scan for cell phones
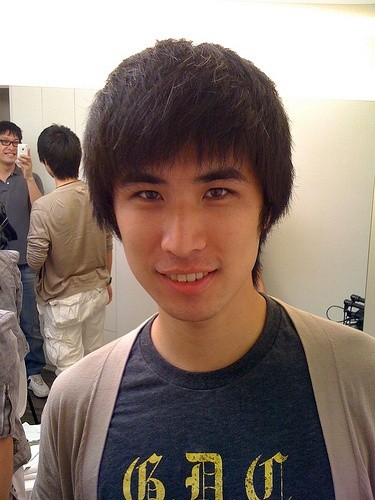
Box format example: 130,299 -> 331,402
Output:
18,144 -> 28,158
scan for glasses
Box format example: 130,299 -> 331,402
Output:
0,139 -> 21,147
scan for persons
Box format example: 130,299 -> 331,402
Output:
26,125 -> 113,376
30,40 -> 375,500
0,228 -> 36,324
0,121 -> 53,399
0,310 -> 31,498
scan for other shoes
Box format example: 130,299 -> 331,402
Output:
27,374 -> 49,397
